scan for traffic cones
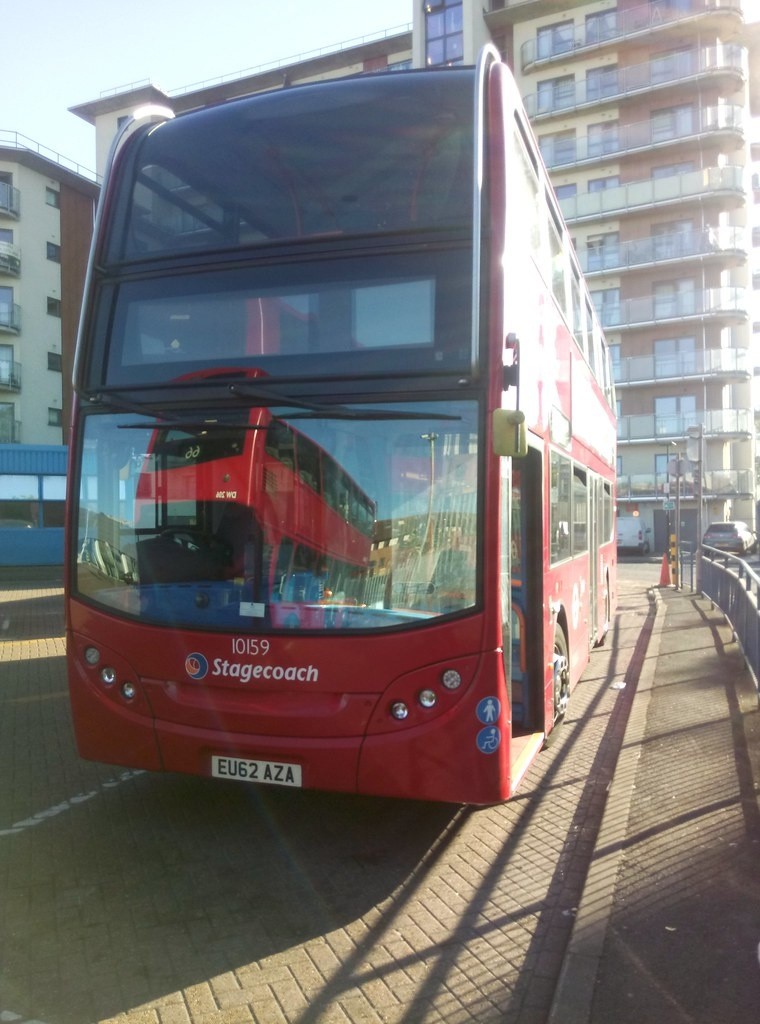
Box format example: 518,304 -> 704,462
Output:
658,549 -> 673,588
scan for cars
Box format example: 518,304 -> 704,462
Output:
702,521 -> 758,557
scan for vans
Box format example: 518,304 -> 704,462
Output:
613,517 -> 652,557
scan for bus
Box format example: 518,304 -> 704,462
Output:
133,365 -> 378,615
216,295 -> 369,355
61,43 -> 620,805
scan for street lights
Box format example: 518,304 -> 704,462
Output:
406,430 -> 443,598
